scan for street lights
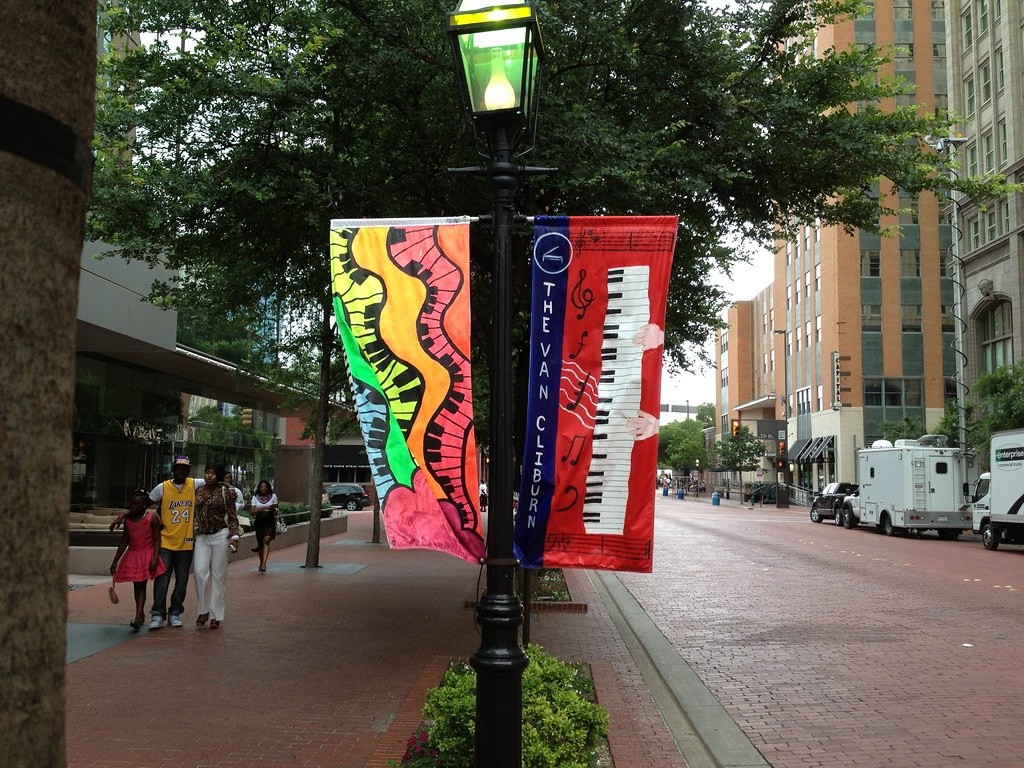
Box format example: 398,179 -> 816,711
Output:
447,0 -> 561,768
774,330 -> 789,509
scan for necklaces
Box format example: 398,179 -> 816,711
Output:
142,513 -> 146,519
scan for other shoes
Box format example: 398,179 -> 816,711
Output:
258,562 -> 267,573
130,616 -> 145,629
196,614 -> 209,626
211,619 -> 220,627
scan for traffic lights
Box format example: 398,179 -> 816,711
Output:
778,461 -> 786,468
779,442 -> 785,456
732,421 -> 740,437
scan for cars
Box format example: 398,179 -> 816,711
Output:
324,483 -> 370,511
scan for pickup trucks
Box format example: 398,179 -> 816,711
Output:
809,483 -> 859,525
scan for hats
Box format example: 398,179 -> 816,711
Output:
173,455 -> 192,466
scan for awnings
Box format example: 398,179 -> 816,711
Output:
788,435 -> 834,464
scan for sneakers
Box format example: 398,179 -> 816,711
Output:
149,615 -> 164,629
168,616 -> 184,627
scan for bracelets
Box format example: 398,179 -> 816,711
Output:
228,487 -> 235,490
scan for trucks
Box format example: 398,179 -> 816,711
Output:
962,426 -> 1024,550
842,434 -> 973,540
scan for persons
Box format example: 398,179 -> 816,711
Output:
479,479 -> 489,512
109,455 -> 244,630
250,480 -> 278,572
656,473 -> 763,511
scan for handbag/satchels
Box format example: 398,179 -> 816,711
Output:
224,513 -> 244,536
275,507 -> 288,536
108,587 -> 119,604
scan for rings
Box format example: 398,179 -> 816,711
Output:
233,497 -> 234,498
